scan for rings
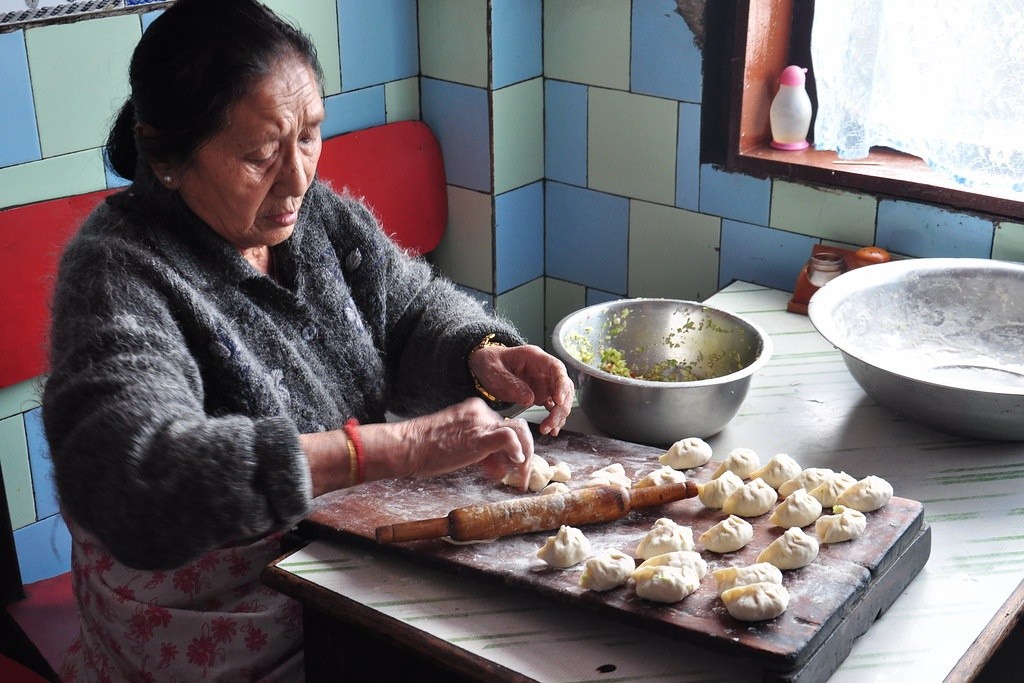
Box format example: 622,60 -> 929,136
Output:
504,416 -> 510,420
545,396 -> 555,409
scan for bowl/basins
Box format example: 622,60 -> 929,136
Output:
808,256 -> 1024,443
551,296 -> 775,447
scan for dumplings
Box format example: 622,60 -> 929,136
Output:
499,437 -> 894,621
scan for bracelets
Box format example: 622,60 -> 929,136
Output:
342,419 -> 364,487
468,333 -> 507,403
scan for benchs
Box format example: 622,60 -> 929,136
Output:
0,120 -> 449,683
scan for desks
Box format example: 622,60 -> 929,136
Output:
265,276 -> 1024,683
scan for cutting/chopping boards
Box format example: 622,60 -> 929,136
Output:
305,418 -> 931,683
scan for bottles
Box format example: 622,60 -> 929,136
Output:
770,61 -> 813,152
808,251 -> 847,288
854,246 -> 890,270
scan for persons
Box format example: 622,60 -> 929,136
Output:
41,0 -> 575,683
